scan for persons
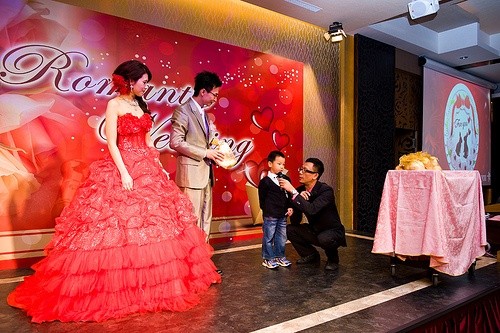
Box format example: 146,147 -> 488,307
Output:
7,60 -> 223,324
258,151 -> 348,270
169,71 -> 225,283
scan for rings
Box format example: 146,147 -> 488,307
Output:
126,185 -> 130,188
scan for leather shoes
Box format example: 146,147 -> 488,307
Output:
297,252 -> 320,264
324,259 -> 339,272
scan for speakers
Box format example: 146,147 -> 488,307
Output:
408,0 -> 440,20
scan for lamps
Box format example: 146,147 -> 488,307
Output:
324,22 -> 347,42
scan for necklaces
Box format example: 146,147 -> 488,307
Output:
117,93 -> 139,111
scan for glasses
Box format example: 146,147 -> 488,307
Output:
208,90 -> 218,98
298,167 -> 317,174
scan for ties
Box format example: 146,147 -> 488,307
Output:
201,109 -> 208,137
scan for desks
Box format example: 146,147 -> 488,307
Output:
371,170 -> 488,285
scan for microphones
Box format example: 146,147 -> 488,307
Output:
278,172 -> 284,191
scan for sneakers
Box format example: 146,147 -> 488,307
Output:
262,258 -> 280,269
274,256 -> 292,267
